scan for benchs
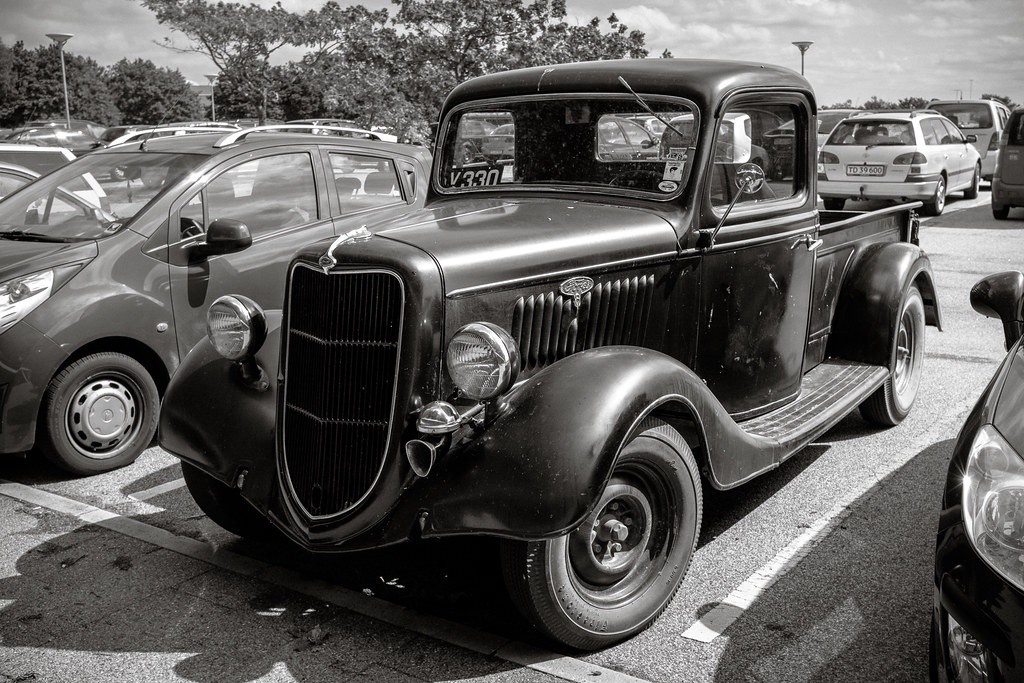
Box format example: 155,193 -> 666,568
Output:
335,173 -> 395,211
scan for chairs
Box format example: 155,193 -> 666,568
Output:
198,172 -> 235,202
854,127 -> 888,140
947,116 -> 958,126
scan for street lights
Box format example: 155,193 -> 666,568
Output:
203,74 -> 220,122
792,41 -> 814,76
45,33 -> 74,129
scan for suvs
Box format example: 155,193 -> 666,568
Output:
816,109 -> 982,217
0,126 -> 431,474
925,97 -> 1011,187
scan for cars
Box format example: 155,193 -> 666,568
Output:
597,116 -> 661,161
761,109 -> 879,182
598,114 -> 622,139
278,119 -> 389,173
620,117 -> 667,139
158,59 -> 943,652
928,269 -> 1024,683
991,105 -> 1024,220
658,108 -> 787,188
0,119 -> 121,225
481,124 -> 515,165
410,119 -> 498,164
92,119 -> 259,190
